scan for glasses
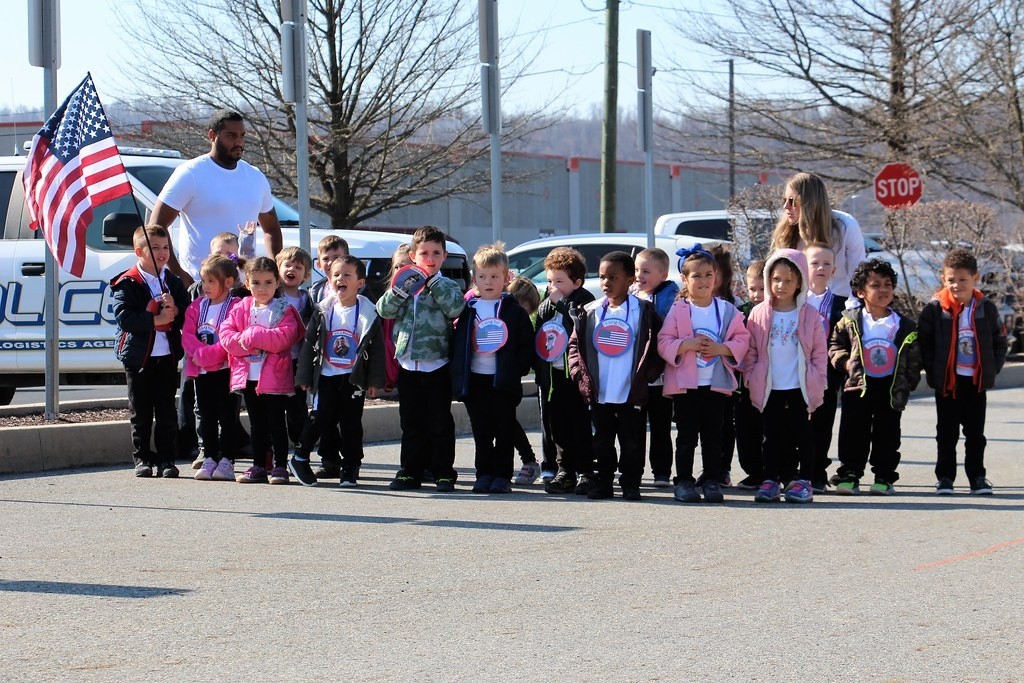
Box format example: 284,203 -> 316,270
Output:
783,197 -> 800,207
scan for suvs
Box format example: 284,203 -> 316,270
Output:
1,141 -> 469,406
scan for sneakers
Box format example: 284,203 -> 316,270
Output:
156,462 -> 178,478
837,479 -> 860,494
870,479 -> 895,494
936,477 -> 954,494
783,480 -> 813,503
190,448 -> 361,486
970,476 -> 993,494
472,469 -> 735,504
134,461 -> 152,476
754,480 -> 781,503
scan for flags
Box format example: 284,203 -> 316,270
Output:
21,74 -> 131,277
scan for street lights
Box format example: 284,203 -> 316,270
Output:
715,59 -> 735,208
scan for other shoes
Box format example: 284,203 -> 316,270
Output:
390,470 -> 422,490
810,479 -> 827,493
437,472 -> 454,491
738,471 -> 758,490
828,472 -> 838,484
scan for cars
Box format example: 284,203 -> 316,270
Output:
508,233 -> 731,300
865,233 -> 1024,351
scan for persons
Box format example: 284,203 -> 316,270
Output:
917,247 -> 1008,494
375,226 -> 466,491
110,225 -> 193,477
454,241 -> 848,503
148,109 -> 284,463
829,259 -> 923,496
235,221 -> 386,486
770,173 -> 867,308
181,232 -> 243,467
220,257 -> 307,485
181,253 -> 244,481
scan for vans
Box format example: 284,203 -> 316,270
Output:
657,209 -> 783,257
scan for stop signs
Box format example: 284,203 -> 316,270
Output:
872,162 -> 922,209
